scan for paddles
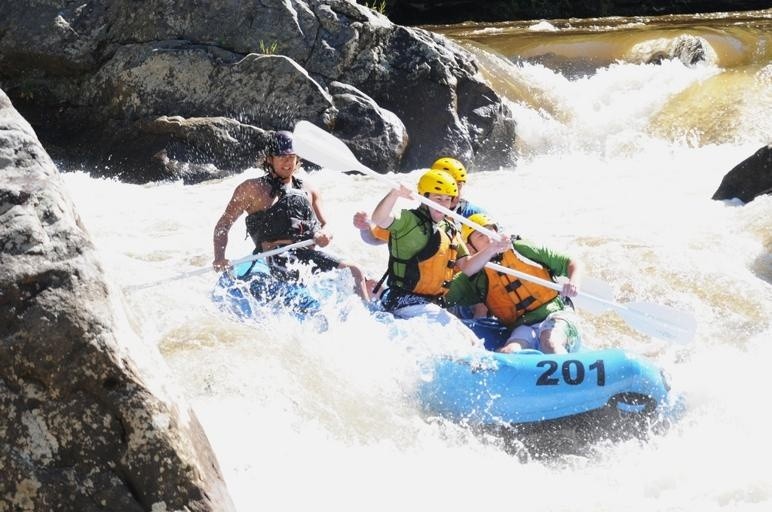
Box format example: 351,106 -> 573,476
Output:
291,120 -> 502,240
121,233 -> 334,294
485,261 -> 706,348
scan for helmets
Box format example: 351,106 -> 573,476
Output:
266,131 -> 295,157
463,212 -> 498,243
418,156 -> 467,198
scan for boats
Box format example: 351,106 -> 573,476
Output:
212,250 -> 664,427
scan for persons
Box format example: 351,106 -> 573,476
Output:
214,129 -> 386,308
371,167 -> 510,319
352,155 -> 486,274
443,213 -> 578,355
